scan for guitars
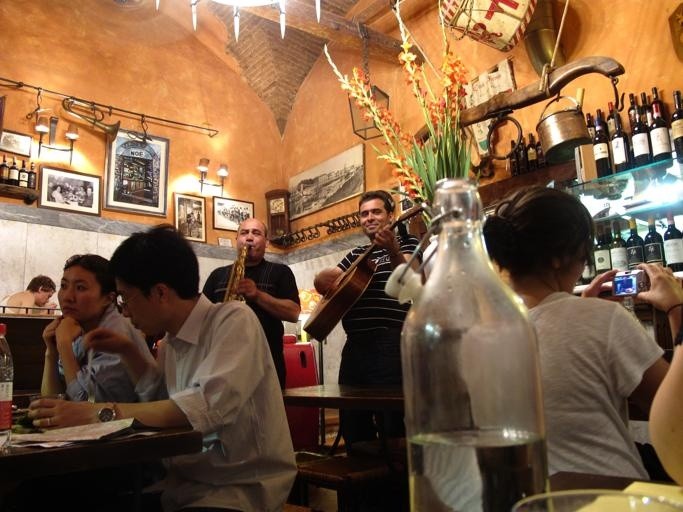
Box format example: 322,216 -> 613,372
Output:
303,200 -> 431,342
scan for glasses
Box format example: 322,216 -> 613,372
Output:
115,292 -> 140,308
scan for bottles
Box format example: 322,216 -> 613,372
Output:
507,138 -> 544,173
584,213 -> 681,273
0,153 -> 37,192
0,323 -> 14,449
584,87 -> 682,176
401,180 -> 552,511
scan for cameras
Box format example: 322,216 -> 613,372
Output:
613,269 -> 646,297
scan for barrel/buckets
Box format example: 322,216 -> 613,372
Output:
536,95 -> 592,166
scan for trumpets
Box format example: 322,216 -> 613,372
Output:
61,97 -> 122,144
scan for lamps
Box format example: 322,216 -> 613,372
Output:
33,115 -> 79,167
341,27 -> 391,139
196,157 -> 229,197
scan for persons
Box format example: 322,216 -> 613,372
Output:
1,275 -> 57,315
485,186 -> 683,511
619,277 -> 632,292
179,199 -> 202,237
47,175 -> 94,208
28,223 -> 297,511
203,218 -> 301,391
649,328 -> 683,488
314,190 -> 423,512
217,205 -> 250,225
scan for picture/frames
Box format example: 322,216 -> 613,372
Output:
102,125 -> 170,217
173,193 -> 206,243
287,141 -> 366,222
0,128 -> 33,157
211,195 -> 253,232
37,164 -> 101,217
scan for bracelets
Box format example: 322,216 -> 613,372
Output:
665,303 -> 682,312
673,327 -> 683,343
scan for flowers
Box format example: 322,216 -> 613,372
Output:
319,5 -> 479,229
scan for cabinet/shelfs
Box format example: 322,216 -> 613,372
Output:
565,154 -> 683,297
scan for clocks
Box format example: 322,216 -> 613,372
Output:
264,188 -> 290,241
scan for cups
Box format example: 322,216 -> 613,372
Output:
29,391 -> 67,431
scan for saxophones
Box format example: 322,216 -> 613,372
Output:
223,243 -> 251,304
